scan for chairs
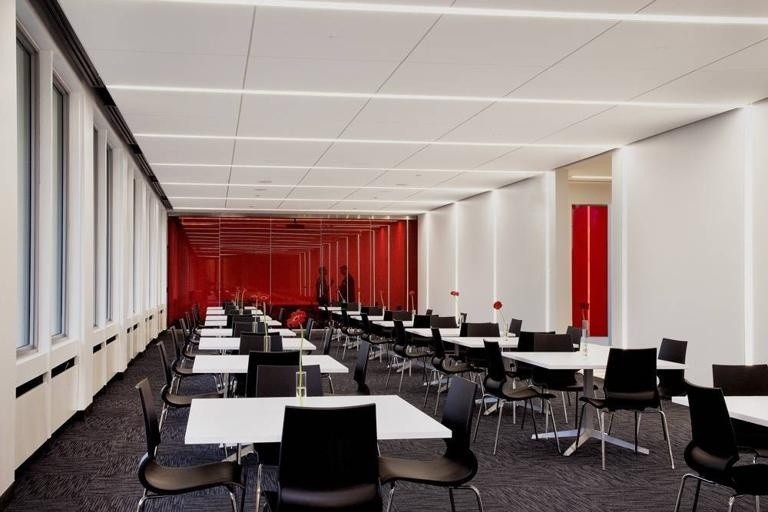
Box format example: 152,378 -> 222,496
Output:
244,363 -> 324,511
135,376 -> 251,511
153,302 -> 688,456
674,383 -> 767,512
575,347 -> 676,471
711,362 -> 767,464
378,374 -> 485,512
255,403 -> 383,511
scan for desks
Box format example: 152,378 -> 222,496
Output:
184,394 -> 454,467
722,395 -> 768,428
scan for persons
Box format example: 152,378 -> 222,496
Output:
315,266 -> 333,306
336,265 -> 356,303
